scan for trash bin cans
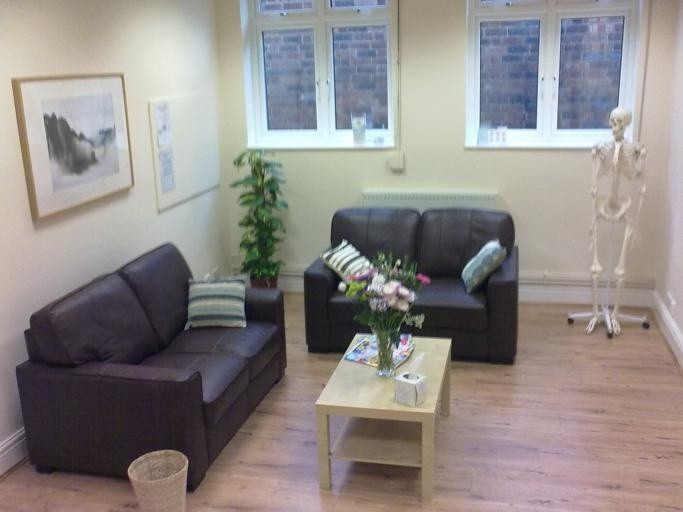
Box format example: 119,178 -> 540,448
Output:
127,450 -> 189,512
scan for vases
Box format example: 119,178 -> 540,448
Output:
372,329 -> 400,377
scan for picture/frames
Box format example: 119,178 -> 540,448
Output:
11,70 -> 136,221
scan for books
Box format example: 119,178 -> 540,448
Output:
344,333 -> 415,370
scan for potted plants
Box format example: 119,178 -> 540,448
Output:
228,147 -> 285,290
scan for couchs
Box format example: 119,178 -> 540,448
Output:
304,206 -> 518,364
15,287 -> 287,494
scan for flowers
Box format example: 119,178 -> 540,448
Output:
337,251 -> 432,348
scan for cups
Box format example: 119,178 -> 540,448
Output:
349,111 -> 367,145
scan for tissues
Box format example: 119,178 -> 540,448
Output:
393,353 -> 428,407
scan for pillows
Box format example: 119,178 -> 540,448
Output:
461,238 -> 508,294
184,273 -> 248,331
119,242 -> 194,349
320,237 -> 372,285
29,272 -> 162,367
418,208 -> 516,278
330,208 -> 422,273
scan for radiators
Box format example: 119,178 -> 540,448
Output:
362,188 -> 499,212
